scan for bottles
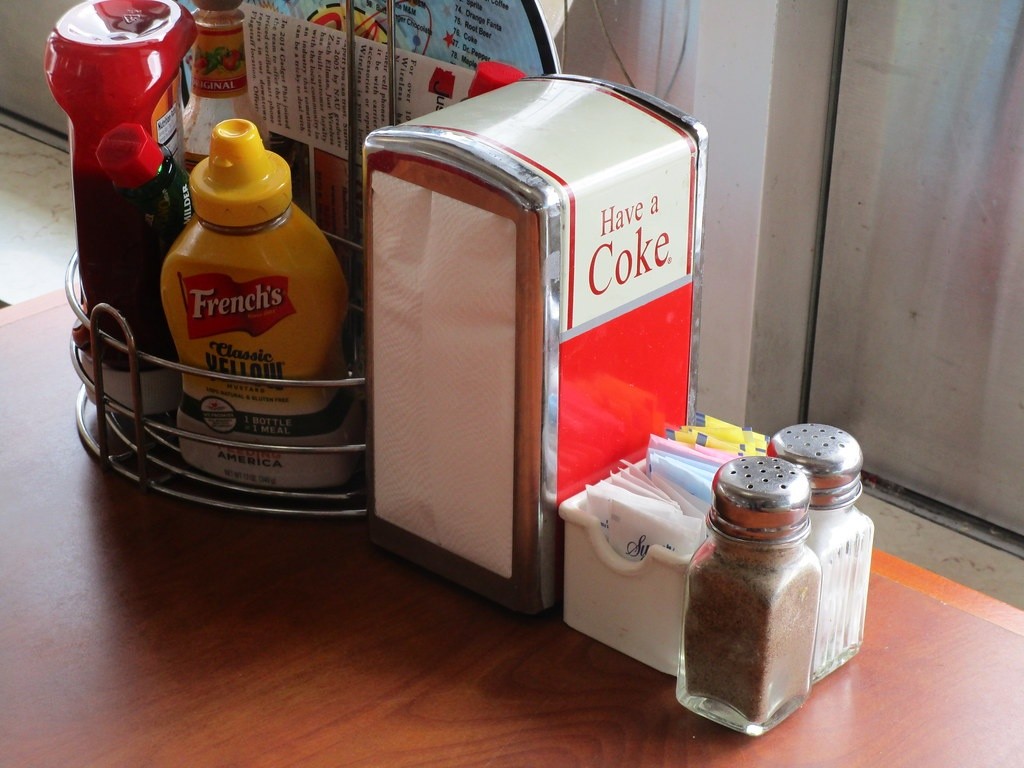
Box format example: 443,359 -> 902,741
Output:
182,0 -> 269,173
677,454 -> 823,734
44,0 -> 196,417
771,424 -> 875,680
160,118 -> 360,493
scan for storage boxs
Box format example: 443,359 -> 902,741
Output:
560,457 -> 688,676
363,72 -> 710,623
